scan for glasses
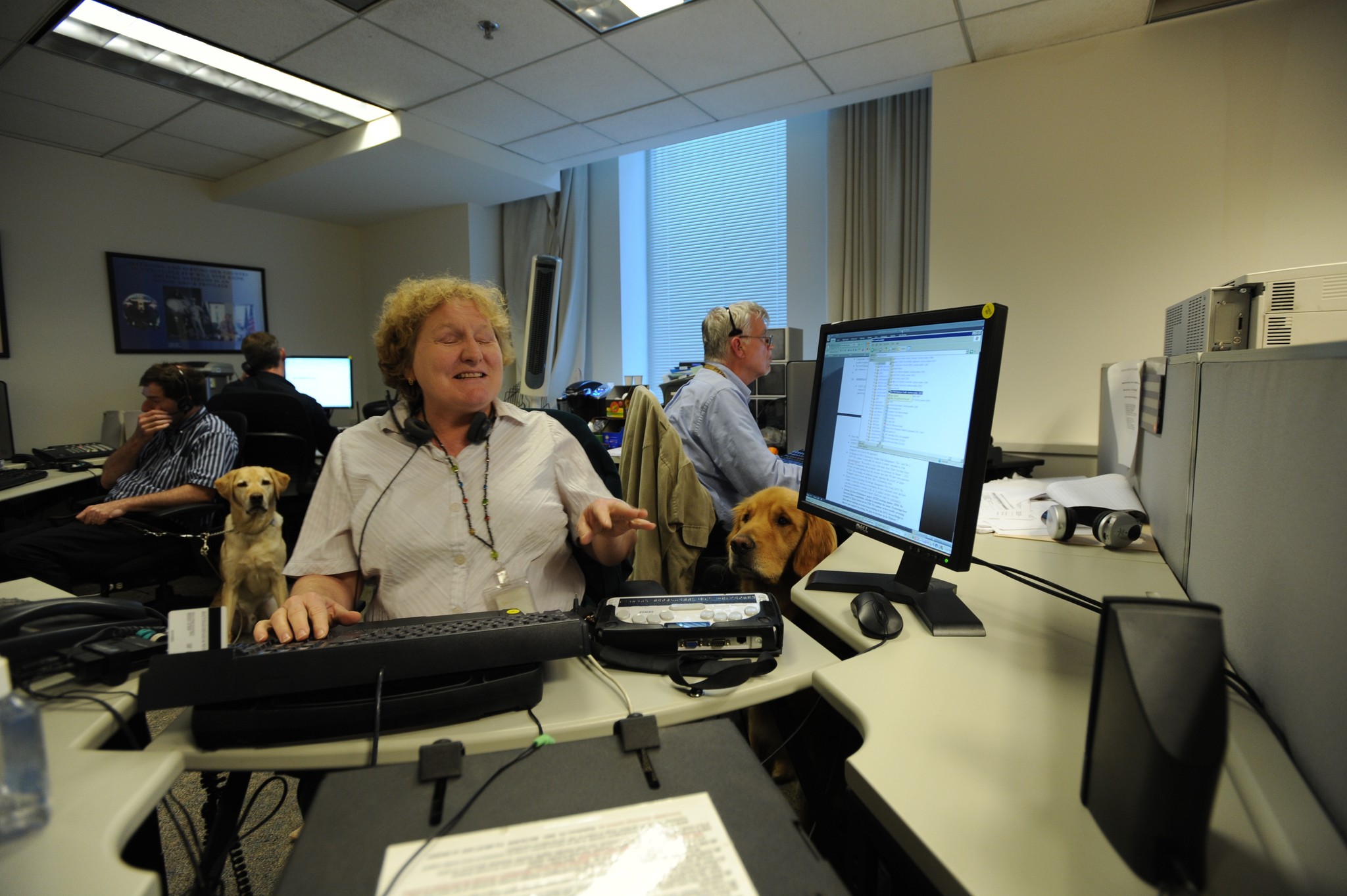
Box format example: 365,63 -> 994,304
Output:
739,334 -> 774,347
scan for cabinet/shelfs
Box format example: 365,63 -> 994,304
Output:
747,327 -> 803,430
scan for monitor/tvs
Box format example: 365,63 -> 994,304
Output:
795,302 -> 1009,637
283,355 -> 354,421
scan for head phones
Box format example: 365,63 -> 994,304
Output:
1040,504 -> 1142,548
384,390 -> 498,447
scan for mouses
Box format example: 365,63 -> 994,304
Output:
851,591 -> 904,641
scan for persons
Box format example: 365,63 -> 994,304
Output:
661,299 -> 804,593
253,274 -> 655,646
207,330 -> 340,554
219,314 -> 237,342
173,297 -> 191,342
187,297 -> 208,341
0,362 -> 238,610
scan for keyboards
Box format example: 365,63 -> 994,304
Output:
0,468 -> 48,491
134,611 -> 590,713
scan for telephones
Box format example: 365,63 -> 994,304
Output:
10,442 -> 115,470
1,596 -> 168,667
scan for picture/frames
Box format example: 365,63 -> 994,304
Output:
105,251 -> 269,353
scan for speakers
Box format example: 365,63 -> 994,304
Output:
1077,596 -> 1226,894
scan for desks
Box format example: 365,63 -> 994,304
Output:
0,476 -> 1345,896
0,457 -> 111,503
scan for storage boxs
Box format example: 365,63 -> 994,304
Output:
587,384 -> 650,450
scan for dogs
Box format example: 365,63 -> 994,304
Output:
210,465 -> 291,645
726,486 -> 837,626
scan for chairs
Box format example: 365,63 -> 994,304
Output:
71,387 -> 739,614
